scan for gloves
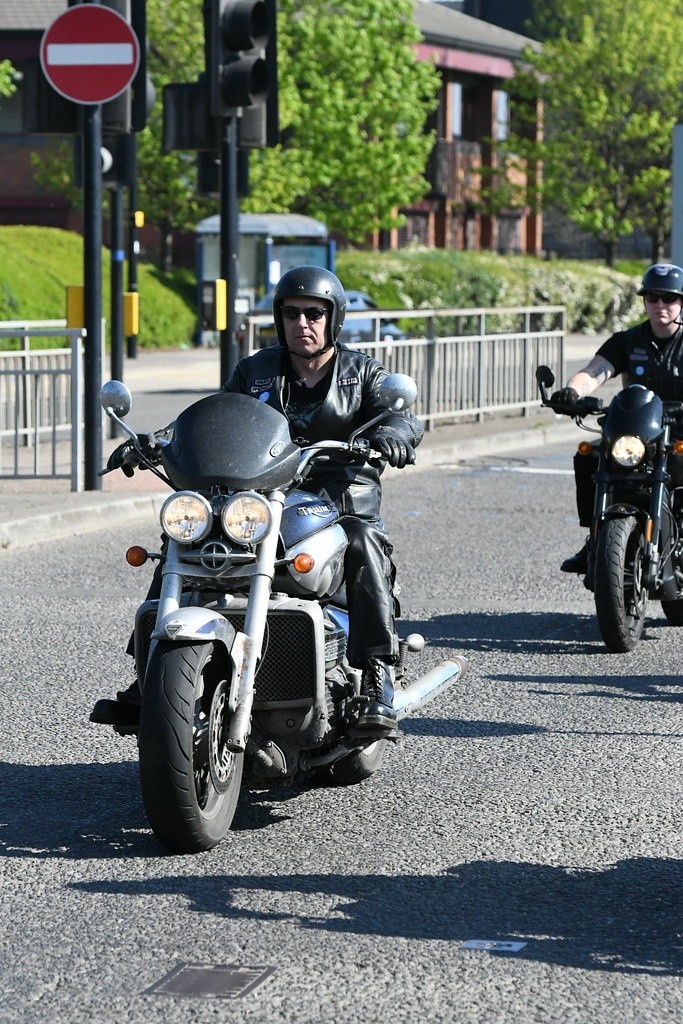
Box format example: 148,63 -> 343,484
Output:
551,387 -> 579,419
107,433 -> 160,477
368,425 -> 416,468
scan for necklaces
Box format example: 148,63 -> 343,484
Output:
641,320 -> 683,377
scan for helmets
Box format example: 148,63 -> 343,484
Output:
272,266 -> 347,346
637,264 -> 683,296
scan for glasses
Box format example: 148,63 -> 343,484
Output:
279,305 -> 331,320
644,293 -> 680,304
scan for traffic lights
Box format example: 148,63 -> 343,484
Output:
222,0 -> 276,109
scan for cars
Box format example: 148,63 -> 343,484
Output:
234,288 -> 406,361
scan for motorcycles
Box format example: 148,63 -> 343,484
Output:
94,381 -> 468,855
534,365 -> 683,654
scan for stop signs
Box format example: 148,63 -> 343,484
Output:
40,4 -> 141,106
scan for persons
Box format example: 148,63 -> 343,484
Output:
105,263 -> 425,730
549,262 -> 683,575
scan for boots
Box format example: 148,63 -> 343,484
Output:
560,536 -> 592,574
356,659 -> 397,728
89,678 -> 144,723
